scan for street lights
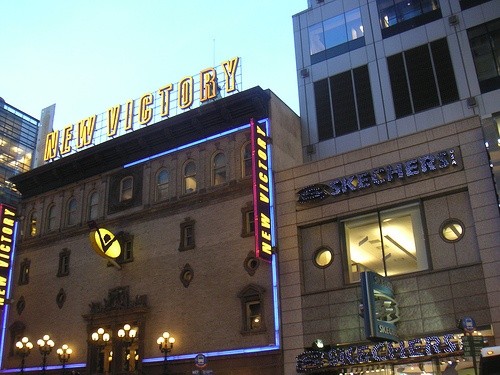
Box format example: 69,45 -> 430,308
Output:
117,324 -> 138,375
91,327 -> 112,375
56,344 -> 72,375
155,331 -> 175,375
16,337 -> 34,375
36,334 -> 55,375
